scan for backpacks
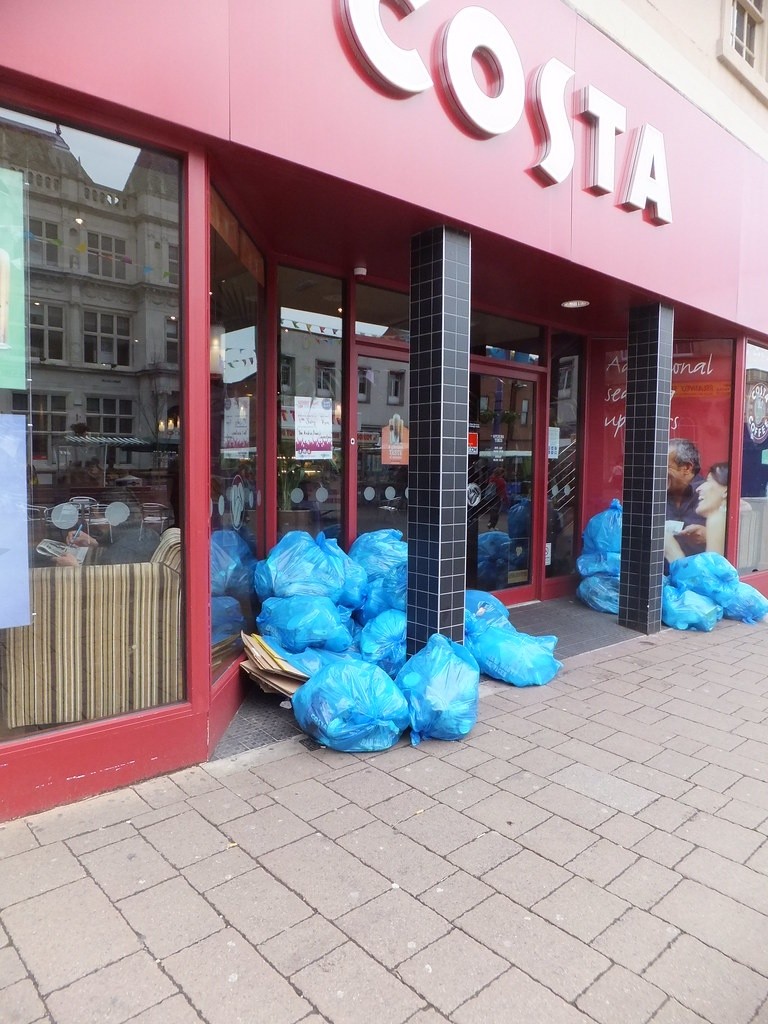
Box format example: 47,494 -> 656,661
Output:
485,478 -> 503,497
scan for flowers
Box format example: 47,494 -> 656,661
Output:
231,446 -> 339,509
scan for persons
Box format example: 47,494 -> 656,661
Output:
50,487 -> 162,566
663,462 -> 752,563
166,458 -> 179,527
664,438 -> 707,577
487,467 -> 506,531
512,480 -> 532,503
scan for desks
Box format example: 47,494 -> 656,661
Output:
69,501 -> 97,531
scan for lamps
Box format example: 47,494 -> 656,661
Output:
38,356 -> 46,364
110,363 -> 117,370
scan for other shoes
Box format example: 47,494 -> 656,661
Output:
488,522 -> 499,532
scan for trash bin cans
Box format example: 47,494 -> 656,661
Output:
545,510 -> 558,578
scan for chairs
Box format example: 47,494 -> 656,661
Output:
377,497 -> 403,524
16,497 -> 114,545
138,502 -> 172,543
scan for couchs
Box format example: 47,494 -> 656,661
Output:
6,527 -> 185,729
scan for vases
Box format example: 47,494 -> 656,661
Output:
242,510 -> 321,544
